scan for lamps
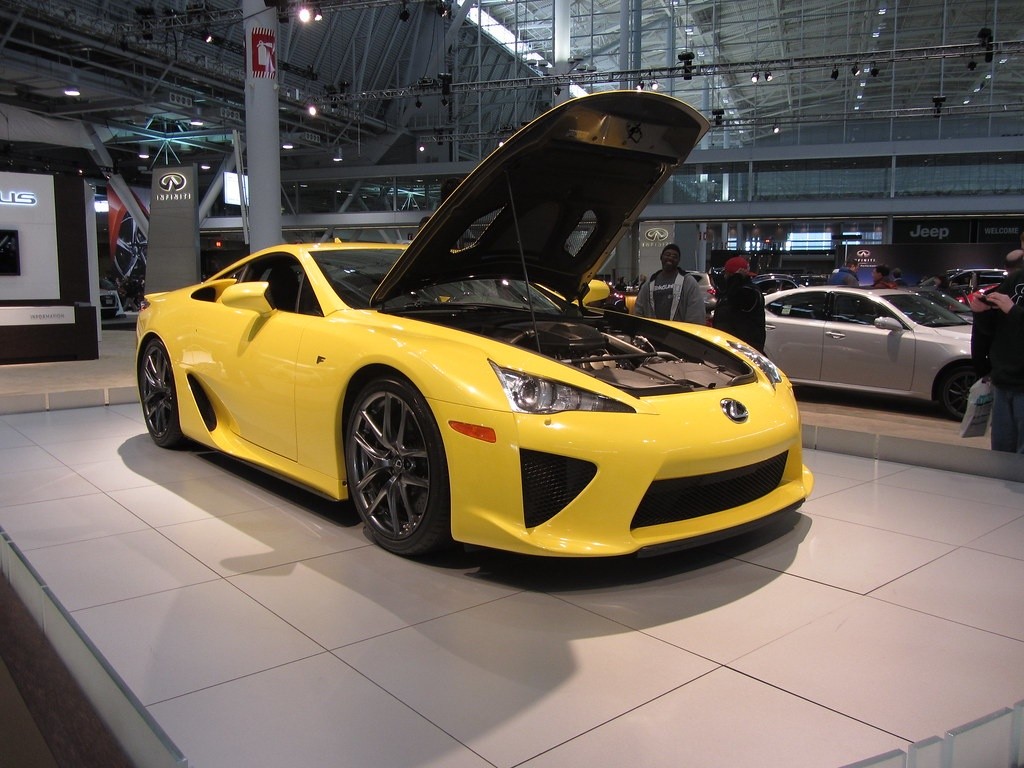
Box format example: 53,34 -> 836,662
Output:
0,0 -> 1023,171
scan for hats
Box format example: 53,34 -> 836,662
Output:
725,257 -> 757,276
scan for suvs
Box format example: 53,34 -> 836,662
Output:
582,281 -> 628,312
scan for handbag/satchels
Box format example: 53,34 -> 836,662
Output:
959,378 -> 995,438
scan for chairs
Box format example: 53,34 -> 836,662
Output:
266,266 -> 307,314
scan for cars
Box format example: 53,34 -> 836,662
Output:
752,274 -> 829,294
948,283 -> 1001,313
131,87 -> 817,560
919,268 -> 1008,291
760,283 -> 990,423
685,270 -> 717,312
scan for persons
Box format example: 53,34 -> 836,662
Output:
935,272 -> 949,288
634,244 -> 706,325
713,258 -> 766,352
872,264 -> 908,290
828,259 -> 859,285
969,232 -> 1024,455
